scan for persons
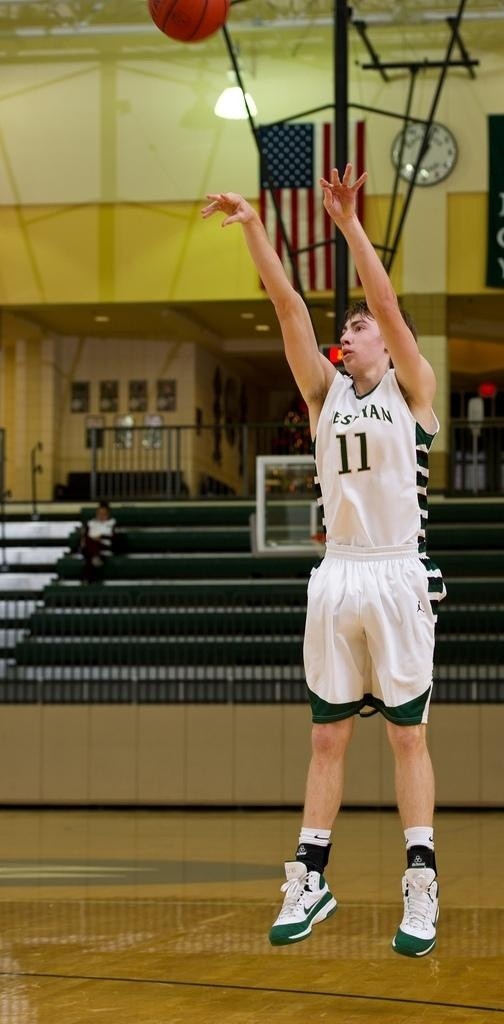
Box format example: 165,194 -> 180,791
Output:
200,161 -> 453,956
84,497 -> 121,580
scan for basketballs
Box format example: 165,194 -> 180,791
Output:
147,0 -> 230,44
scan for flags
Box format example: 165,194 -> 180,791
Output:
254,120 -> 365,288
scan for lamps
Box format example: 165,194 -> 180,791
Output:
215,39 -> 258,119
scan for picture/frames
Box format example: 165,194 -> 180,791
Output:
71,379 -> 175,449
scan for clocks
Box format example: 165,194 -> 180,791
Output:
390,121 -> 458,185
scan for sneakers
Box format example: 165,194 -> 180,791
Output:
390,866 -> 441,959
269,859 -> 339,947
